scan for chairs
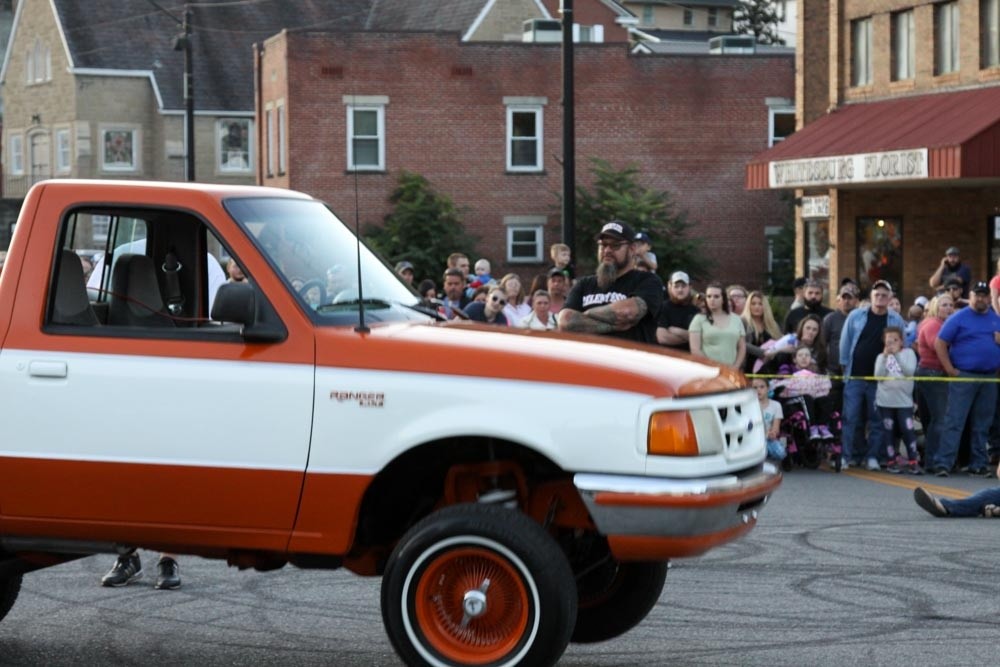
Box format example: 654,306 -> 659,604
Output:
49,248 -> 176,329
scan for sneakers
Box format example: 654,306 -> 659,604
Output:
154,556 -> 181,589
103,554 -> 144,587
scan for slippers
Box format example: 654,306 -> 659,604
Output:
914,487 -> 948,517
982,502 -> 998,517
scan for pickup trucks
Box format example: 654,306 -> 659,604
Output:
0,179 -> 786,667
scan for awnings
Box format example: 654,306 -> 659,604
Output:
743,83 -> 1000,191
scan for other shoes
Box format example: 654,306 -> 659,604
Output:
808,425 -> 821,440
829,457 -> 850,469
819,425 -> 835,439
865,457 -> 881,471
968,467 -> 992,478
908,464 -> 924,475
935,467 -> 949,476
887,463 -> 904,473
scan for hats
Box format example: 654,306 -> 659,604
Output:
394,261 -> 414,274
594,222 -> 635,241
972,281 -> 991,294
547,268 -> 564,279
914,296 -> 929,308
946,245 -> 960,255
672,271 -> 690,285
633,232 -> 651,244
842,287 -> 854,299
873,280 -> 892,290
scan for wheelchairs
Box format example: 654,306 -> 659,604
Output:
773,385 -> 845,474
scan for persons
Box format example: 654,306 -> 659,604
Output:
633,231 -> 1000,478
86,238 -> 226,589
226,243 -> 578,332
0,251 -> 103,284
558,220 -> 663,345
914,463 -> 1000,518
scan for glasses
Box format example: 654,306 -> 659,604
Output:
946,287 -> 958,291
597,242 -> 628,250
493,296 -> 505,305
